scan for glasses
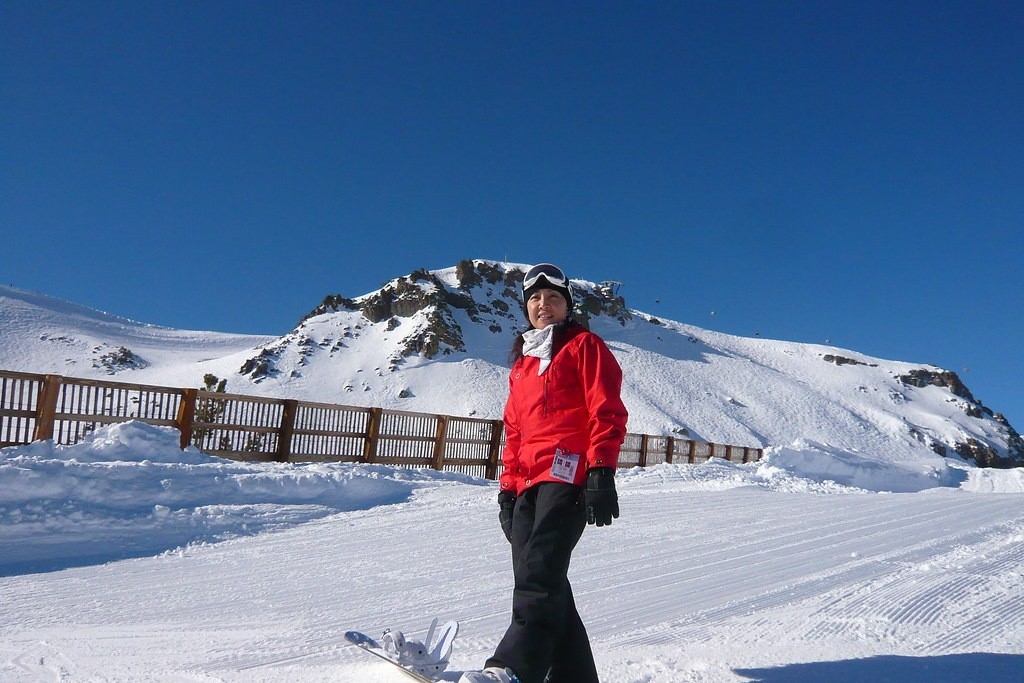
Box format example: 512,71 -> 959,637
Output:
523,263 -> 572,298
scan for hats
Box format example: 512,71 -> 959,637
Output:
522,273 -> 573,323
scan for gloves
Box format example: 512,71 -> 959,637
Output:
578,468 -> 619,527
498,492 -> 517,544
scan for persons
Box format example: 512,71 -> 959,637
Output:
465,263 -> 628,683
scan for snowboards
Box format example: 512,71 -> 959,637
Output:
343,617 -> 521,683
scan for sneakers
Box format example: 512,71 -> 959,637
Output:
458,667 -> 520,683
